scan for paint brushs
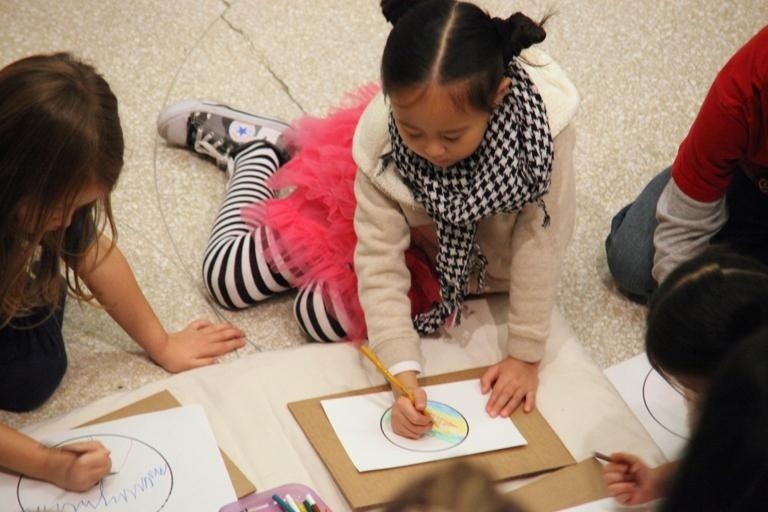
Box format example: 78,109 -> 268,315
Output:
271,493 -> 321,512
360,344 -> 442,432
38,443 -> 86,456
593,450 -> 629,469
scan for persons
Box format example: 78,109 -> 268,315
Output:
0,53 -> 246,496
603,23 -> 766,303
157,0 -> 589,440
602,251 -> 765,512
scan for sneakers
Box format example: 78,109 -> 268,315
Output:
156,99 -> 300,177
